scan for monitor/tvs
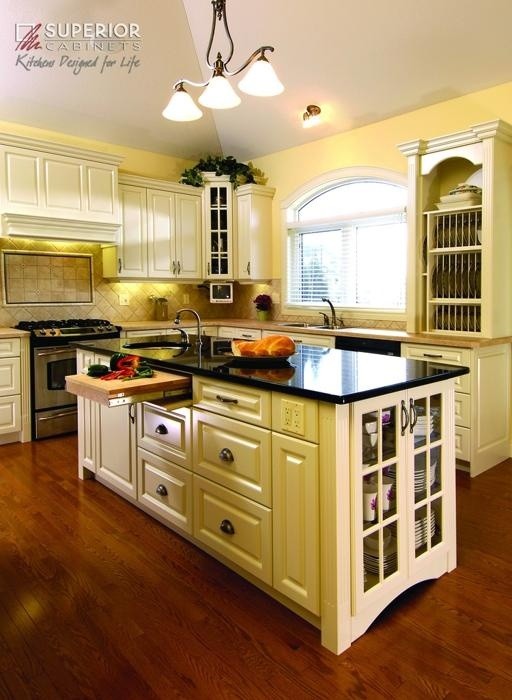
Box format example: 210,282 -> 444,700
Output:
209,283 -> 234,303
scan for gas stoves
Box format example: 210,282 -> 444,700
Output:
9,318 -> 119,342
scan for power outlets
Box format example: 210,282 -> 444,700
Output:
119,293 -> 129,306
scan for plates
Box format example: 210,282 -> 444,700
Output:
448,184 -> 483,195
421,215 -> 481,332
217,347 -> 295,362
362,394 -> 440,592
219,360 -> 298,369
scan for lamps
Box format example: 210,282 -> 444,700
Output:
159,1 -> 285,124
303,102 -> 322,126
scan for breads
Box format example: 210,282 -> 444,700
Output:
230,334 -> 295,357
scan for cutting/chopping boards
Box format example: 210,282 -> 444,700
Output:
64,365 -> 192,395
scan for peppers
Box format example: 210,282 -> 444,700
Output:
88,352 -> 154,382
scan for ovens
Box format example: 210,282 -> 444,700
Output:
33,344 -> 76,442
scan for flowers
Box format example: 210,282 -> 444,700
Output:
253,294 -> 272,310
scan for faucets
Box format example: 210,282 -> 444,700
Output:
195,342 -> 203,368
174,308 -> 202,343
322,296 -> 337,326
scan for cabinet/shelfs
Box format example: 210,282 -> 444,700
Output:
147,188 -> 203,283
102,184 -> 148,282
234,182 -> 278,284
0,333 -> 31,447
198,172 -> 234,280
126,326 -> 218,339
397,119 -> 512,340
317,376 -> 455,655
0,132 -> 126,242
218,326 -> 336,348
400,343 -> 512,480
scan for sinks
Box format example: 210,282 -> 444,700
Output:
316,325 -> 356,330
121,341 -> 193,350
276,322 -> 332,328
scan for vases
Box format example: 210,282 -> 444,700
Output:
257,308 -> 268,321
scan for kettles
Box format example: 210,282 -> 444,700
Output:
155,298 -> 169,321
